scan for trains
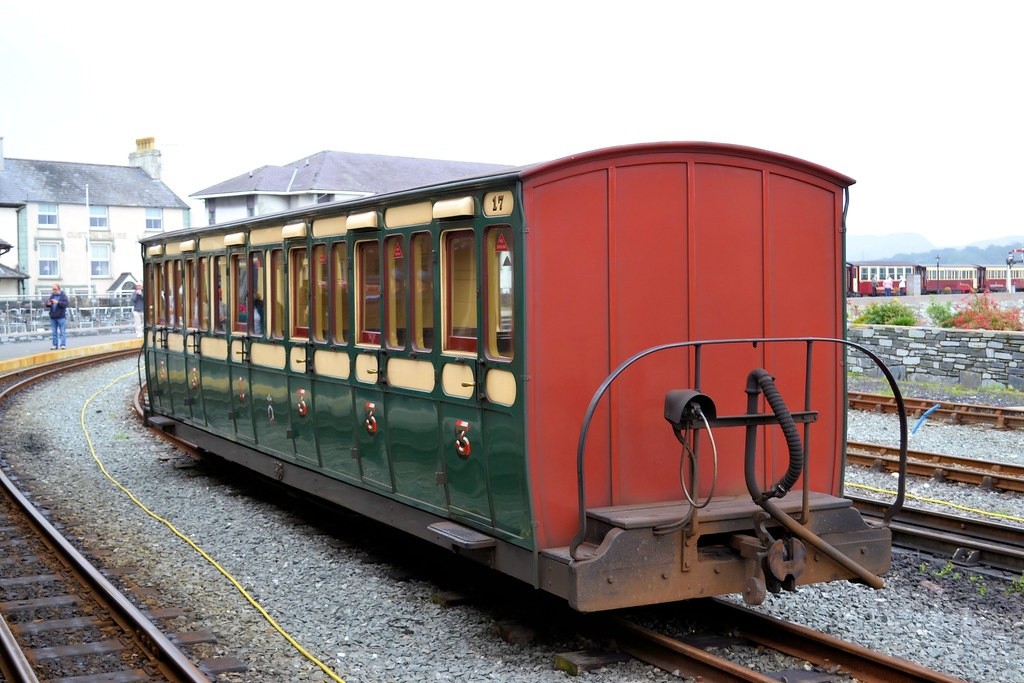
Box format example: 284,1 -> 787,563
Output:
843,263 -> 1024,294
137,143 -> 914,610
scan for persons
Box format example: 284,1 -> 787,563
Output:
239,264 -> 265,335
131,282 -> 144,337
883,274 -> 893,296
45,284 -> 69,350
872,275 -> 878,297
192,285 -> 247,332
898,275 -> 906,295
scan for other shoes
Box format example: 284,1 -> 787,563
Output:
51,346 -> 59,350
61,345 -> 65,349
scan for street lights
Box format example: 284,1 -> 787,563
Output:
934,254 -> 941,294
